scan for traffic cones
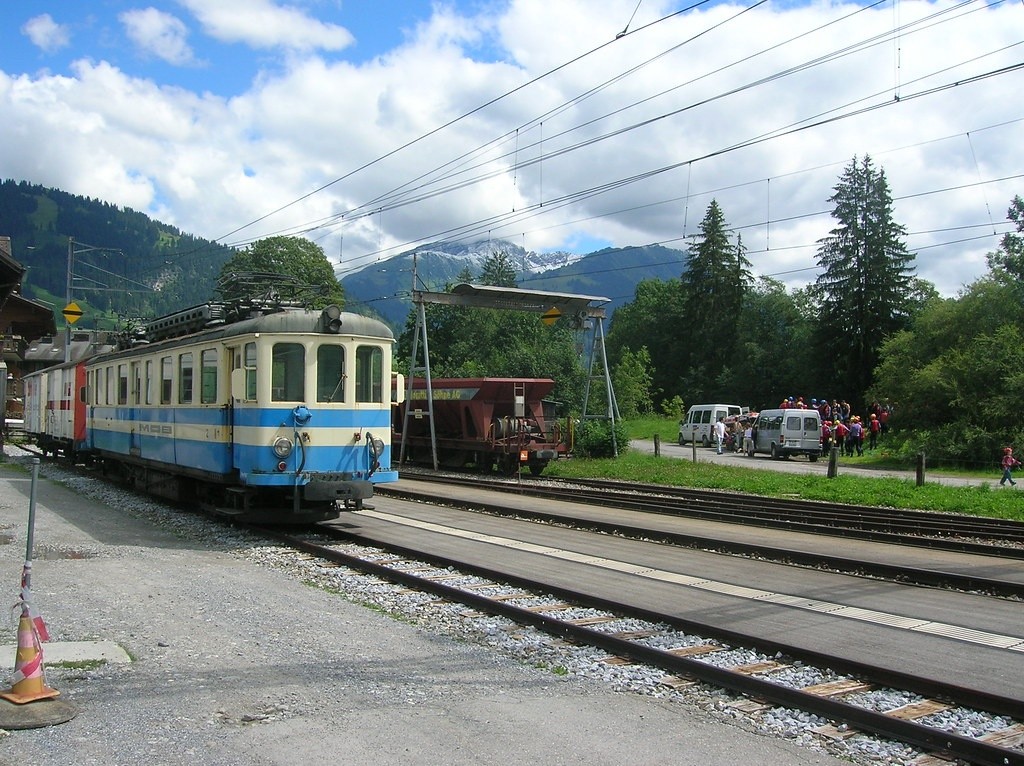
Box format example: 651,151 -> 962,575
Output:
0,605 -> 60,705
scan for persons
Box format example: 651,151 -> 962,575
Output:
1000,448 -> 1021,486
712,416 -> 754,457
780,396 -> 900,457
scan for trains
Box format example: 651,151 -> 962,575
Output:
327,371 -> 574,477
20,296 -> 405,526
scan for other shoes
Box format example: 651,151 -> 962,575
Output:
717,453 -> 723,455
1012,482 -> 1016,486
1000,481 -> 1005,486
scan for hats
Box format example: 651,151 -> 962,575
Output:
821,400 -> 826,404
1004,448 -> 1012,454
783,398 -> 788,403
834,420 -> 840,425
850,415 -> 861,424
826,421 -> 832,426
789,397 -> 793,401
832,399 -> 838,402
799,397 -> 803,401
870,413 -> 876,419
811,398 -> 817,403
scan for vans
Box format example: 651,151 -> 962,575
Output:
746,409 -> 823,462
678,403 -> 750,450
